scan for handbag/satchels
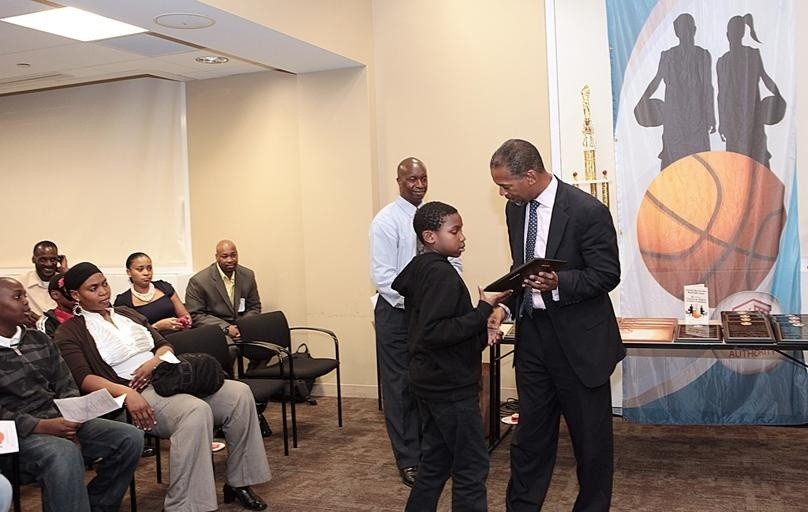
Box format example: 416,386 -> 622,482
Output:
152,352 -> 224,398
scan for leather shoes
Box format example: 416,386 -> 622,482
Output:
259,413 -> 272,436
400,466 -> 417,487
140,447 -> 155,456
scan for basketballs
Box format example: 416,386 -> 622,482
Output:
636,152 -> 787,307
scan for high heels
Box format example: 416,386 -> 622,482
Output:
222,484 -> 267,511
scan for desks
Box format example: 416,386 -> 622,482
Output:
489,321 -> 808,457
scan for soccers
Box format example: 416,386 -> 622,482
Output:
706,291 -> 788,376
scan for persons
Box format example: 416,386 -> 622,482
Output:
488,140 -> 619,512
64,265 -> 272,512
716,14 -> 786,168
37,272 -> 79,337
393,203 -> 513,511
184,240 -> 272,438
636,14 -> 716,170
370,156 -> 462,488
18,241 -> 69,327
114,252 -> 194,339
0,474 -> 15,512
0,275 -> 143,512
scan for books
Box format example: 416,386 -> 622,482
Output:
618,315 -> 808,346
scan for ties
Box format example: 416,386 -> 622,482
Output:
524,201 -> 539,318
414,209 -> 424,255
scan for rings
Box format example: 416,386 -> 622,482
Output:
173,325 -> 175,329
143,378 -> 147,382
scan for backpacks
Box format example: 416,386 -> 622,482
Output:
269,343 -> 317,404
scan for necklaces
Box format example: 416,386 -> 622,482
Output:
130,282 -> 155,302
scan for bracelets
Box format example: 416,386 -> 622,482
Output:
222,326 -> 229,335
